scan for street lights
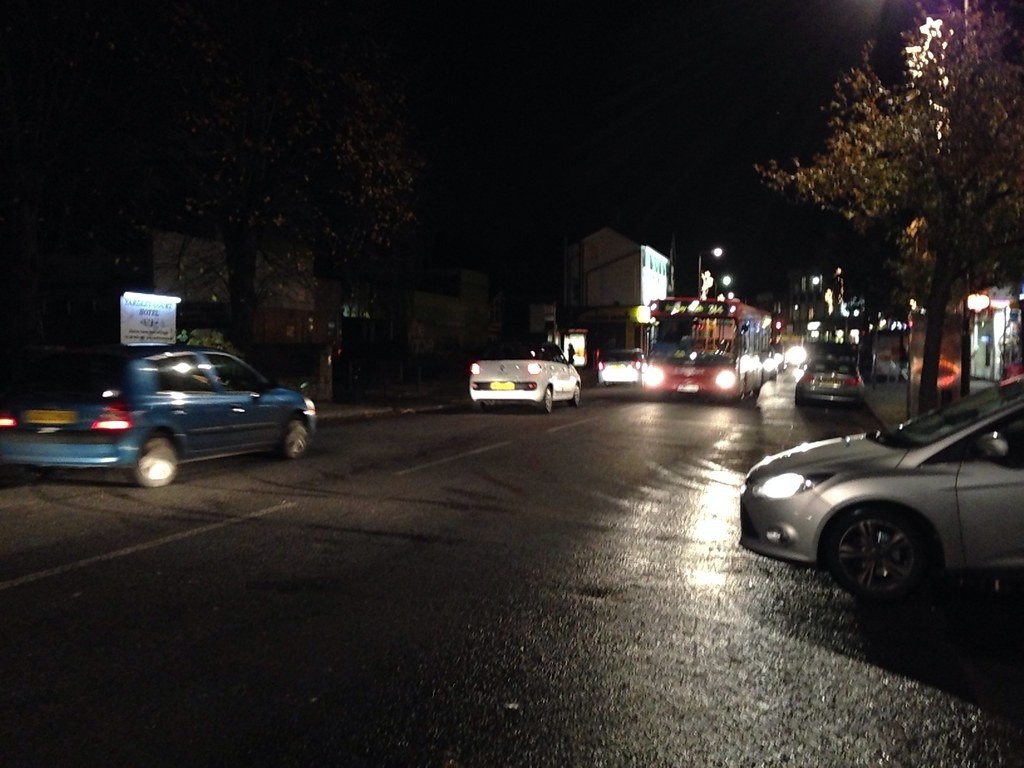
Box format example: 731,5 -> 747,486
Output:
697,246 -> 723,297
715,276 -> 732,297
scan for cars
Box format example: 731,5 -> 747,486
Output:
737,371 -> 1024,607
762,342 -> 807,382
0,342 -> 319,487
793,354 -> 863,413
468,340 -> 584,409
599,347 -> 647,386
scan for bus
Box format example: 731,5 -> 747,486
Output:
639,294 -> 775,400
